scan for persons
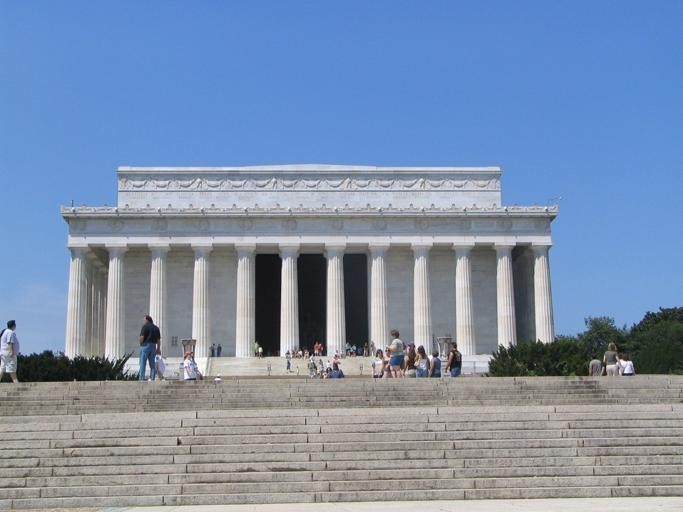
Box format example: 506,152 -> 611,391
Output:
0,319 -> 21,383
138,315 -> 222,380
254,328 -> 462,378
587,342 -> 636,375
